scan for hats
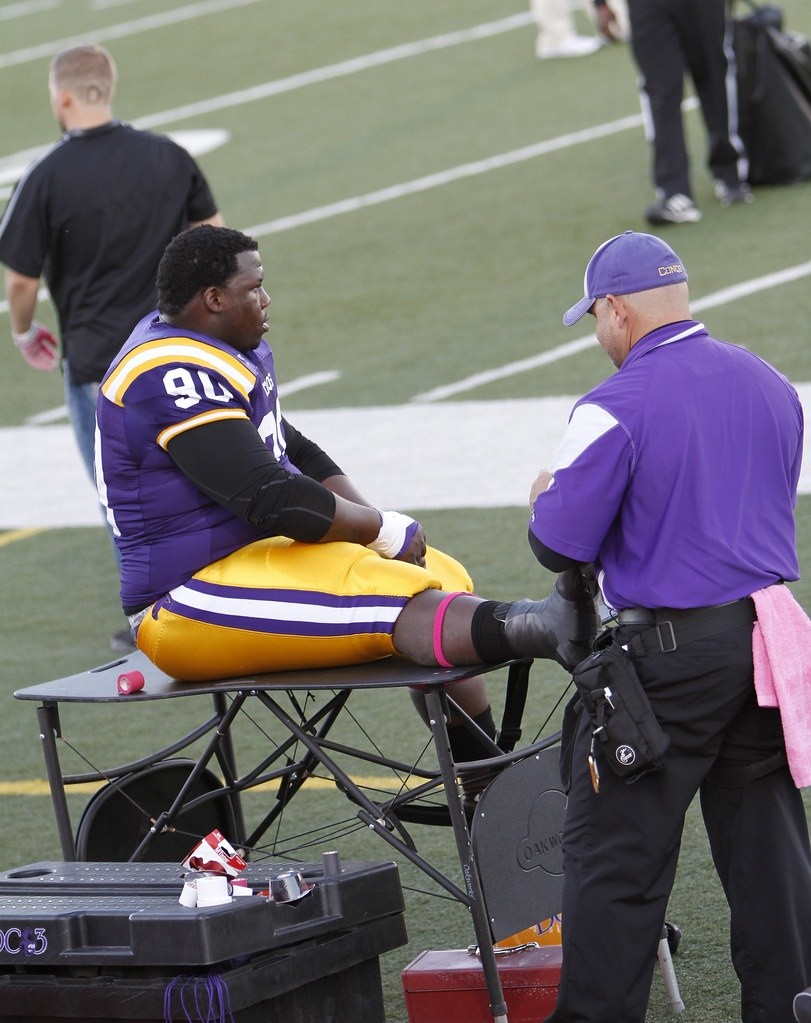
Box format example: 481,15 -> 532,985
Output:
562,229 -> 688,326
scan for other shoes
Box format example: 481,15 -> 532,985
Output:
110,629 -> 135,653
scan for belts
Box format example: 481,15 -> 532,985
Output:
616,604 -> 728,625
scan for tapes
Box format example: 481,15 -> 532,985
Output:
271,869 -> 309,901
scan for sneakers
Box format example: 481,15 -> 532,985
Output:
714,178 -> 752,206
645,188 -> 703,224
536,35 -> 601,59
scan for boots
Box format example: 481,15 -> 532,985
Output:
495,562 -> 603,673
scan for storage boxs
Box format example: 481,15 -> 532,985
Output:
0,861 -> 408,1023
179,828 -> 246,879
400,945 -> 563,1023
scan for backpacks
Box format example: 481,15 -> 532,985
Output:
731,0 -> 811,186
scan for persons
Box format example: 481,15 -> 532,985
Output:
0,45 -> 222,652
526,228 -> 811,1022
591,0 -> 747,226
95,219 -> 681,960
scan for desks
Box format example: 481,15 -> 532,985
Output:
12,647 -> 684,1023
